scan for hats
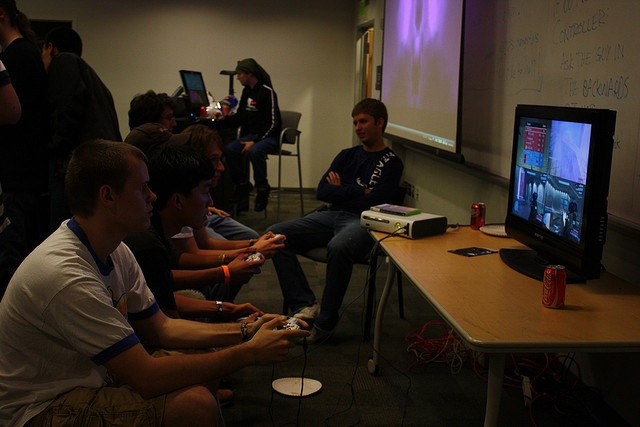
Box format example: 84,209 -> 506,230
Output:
124,123 -> 192,156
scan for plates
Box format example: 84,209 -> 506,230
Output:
479,224 -> 507,237
271,377 -> 323,396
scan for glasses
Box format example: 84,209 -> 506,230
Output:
210,156 -> 225,163
159,115 -> 176,120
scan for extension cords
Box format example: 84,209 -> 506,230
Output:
522,375 -> 533,407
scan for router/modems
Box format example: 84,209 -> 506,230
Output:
358,210 -> 448,239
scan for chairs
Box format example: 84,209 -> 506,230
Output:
239,110 -> 305,219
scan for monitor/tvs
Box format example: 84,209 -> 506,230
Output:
178,69 -> 210,117
498,103 -> 618,284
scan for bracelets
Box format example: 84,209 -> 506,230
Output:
249,238 -> 255,247
218,252 -> 226,265
215,300 -> 223,317
221,265 -> 230,288
240,319 -> 254,343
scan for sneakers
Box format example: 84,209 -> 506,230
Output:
254,182 -> 271,211
217,389 -> 234,405
232,182 -> 253,200
287,300 -> 320,319
305,323 -> 335,343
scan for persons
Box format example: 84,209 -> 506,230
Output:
265,99 -> 404,345
129,89 -> 262,245
2,60 -> 29,300
528,192 -> 545,227
0,0 -> 45,265
220,58 -> 281,212
41,24 -> 123,228
557,202 -> 578,238
126,123 -> 268,302
149,123 -> 287,268
113,145 -> 266,354
1,141 -> 311,426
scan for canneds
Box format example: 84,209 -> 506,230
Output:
470,202 -> 486,231
542,264 -> 566,308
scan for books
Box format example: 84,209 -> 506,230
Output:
370,203 -> 421,216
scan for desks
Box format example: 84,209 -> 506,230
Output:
366,221 -> 637,427
170,113 -> 251,216
